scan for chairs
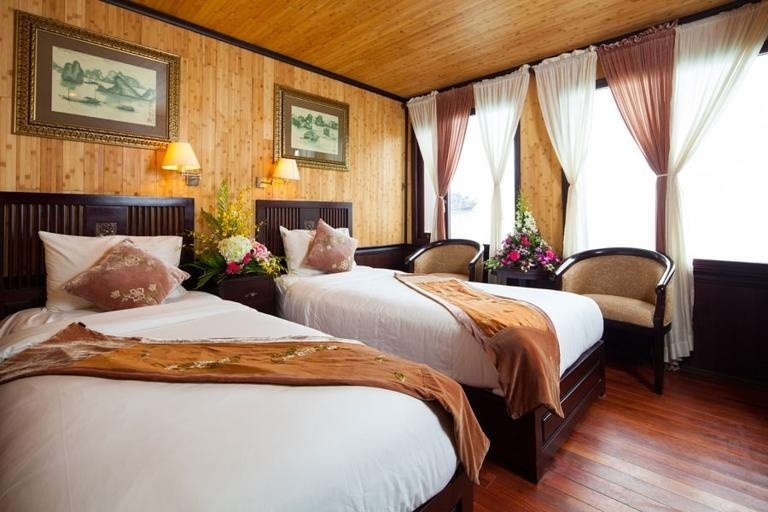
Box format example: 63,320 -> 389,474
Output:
554,246 -> 676,395
405,239 -> 485,282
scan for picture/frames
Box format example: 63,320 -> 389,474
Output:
273,83 -> 350,173
11,9 -> 182,153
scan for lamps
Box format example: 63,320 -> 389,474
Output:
160,140 -> 202,188
256,156 -> 302,189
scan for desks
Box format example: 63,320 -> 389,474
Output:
489,267 -> 555,290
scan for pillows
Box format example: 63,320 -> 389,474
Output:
277,225 -> 359,276
62,238 -> 192,311
38,228 -> 189,313
303,218 -> 359,272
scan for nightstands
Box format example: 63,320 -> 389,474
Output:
209,274 -> 279,317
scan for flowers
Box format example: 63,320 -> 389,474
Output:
482,184 -> 562,281
177,176 -> 298,290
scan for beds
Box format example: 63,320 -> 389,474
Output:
255,199 -> 606,483
0,189 -> 492,512
